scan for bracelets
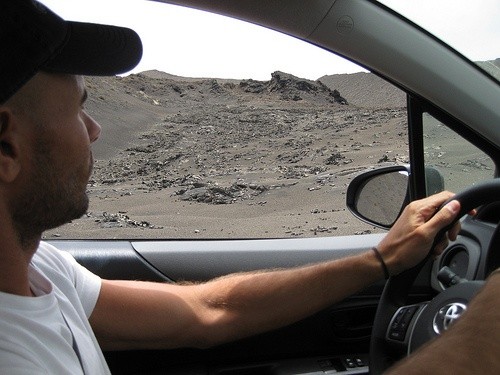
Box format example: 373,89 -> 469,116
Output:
371,246 -> 391,282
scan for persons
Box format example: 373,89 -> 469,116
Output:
0,0 -> 500,374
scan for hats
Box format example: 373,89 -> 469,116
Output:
1,1 -> 142,103
